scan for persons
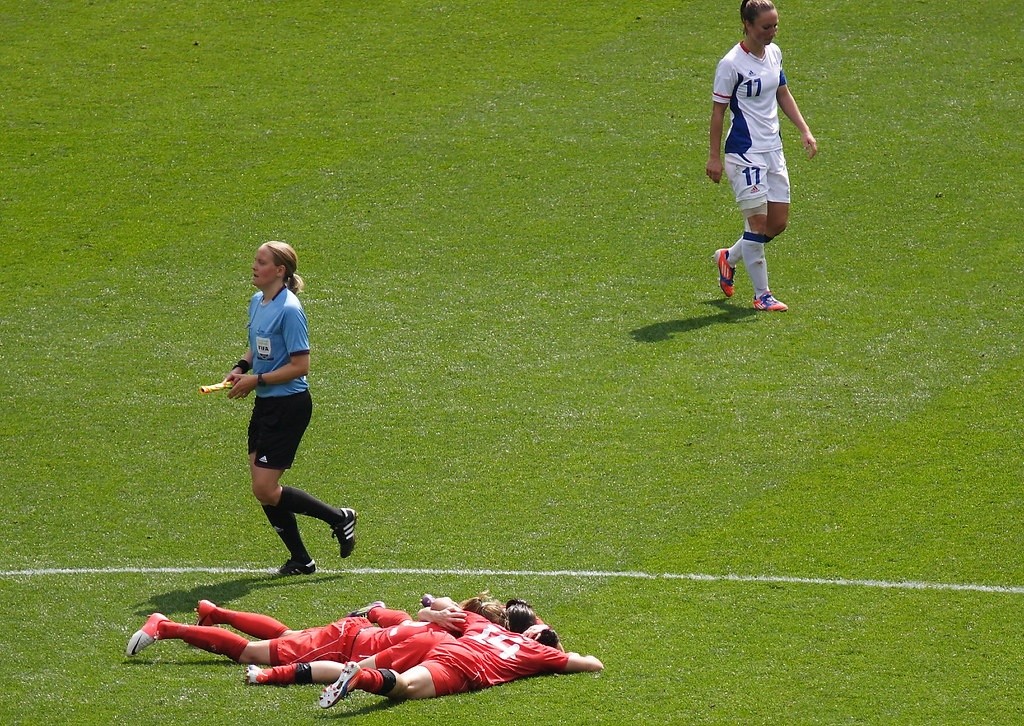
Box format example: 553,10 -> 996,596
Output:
126,595 -> 603,709
706,0 -> 821,311
225,240 -> 356,576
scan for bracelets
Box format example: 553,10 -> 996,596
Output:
258,373 -> 265,387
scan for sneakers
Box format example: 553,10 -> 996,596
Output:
126,613 -> 170,656
245,665 -> 269,684
330,507 -> 357,558
194,599 -> 216,626
714,247 -> 735,297
753,291 -> 789,312
319,661 -> 360,709
270,559 -> 316,577
